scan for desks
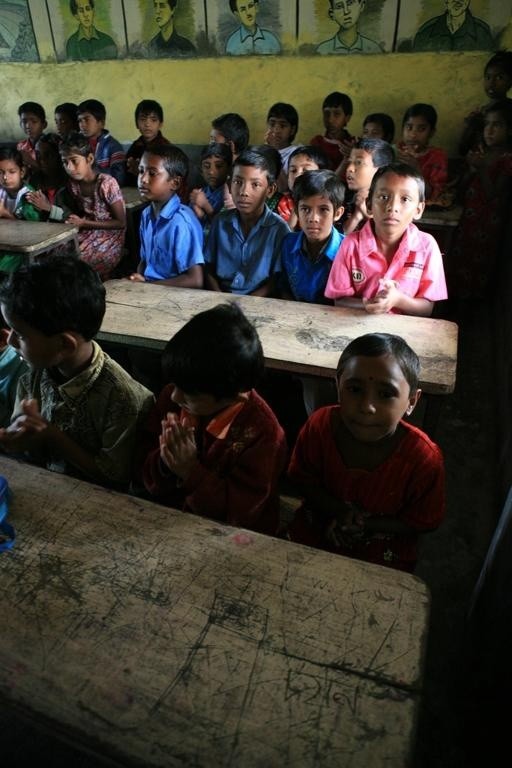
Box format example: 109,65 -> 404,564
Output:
1,456 -> 432,768
413,198 -> 467,232
92,278 -> 460,396
0,218 -> 80,264
121,186 -> 150,253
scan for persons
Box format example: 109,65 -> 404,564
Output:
0,52 -> 510,316
313,0 -> 386,54
66,0 -> 118,61
271,331 -> 446,572
0,246 -> 157,492
142,0 -> 199,57
413,1 -> 496,51
127,301 -> 285,535
225,0 -> 282,55
